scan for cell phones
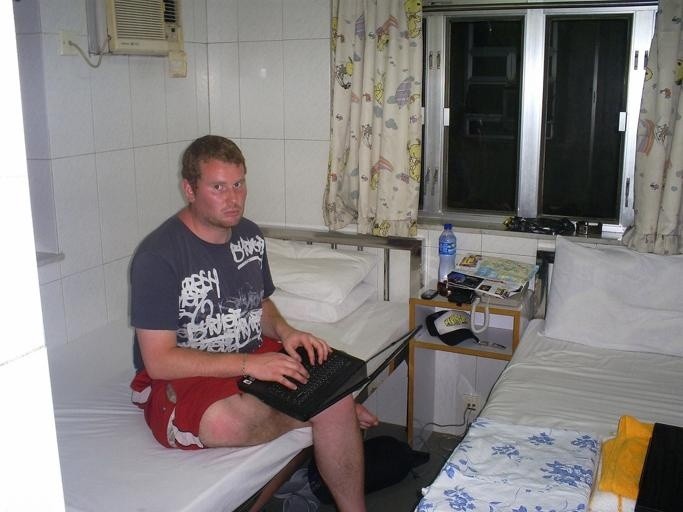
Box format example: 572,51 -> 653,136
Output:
421,289 -> 438,300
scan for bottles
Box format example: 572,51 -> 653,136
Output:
438,224 -> 456,289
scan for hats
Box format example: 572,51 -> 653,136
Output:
425,309 -> 478,348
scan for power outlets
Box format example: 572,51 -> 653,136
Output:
463,393 -> 481,415
58,29 -> 81,57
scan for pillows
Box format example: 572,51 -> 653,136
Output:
266,282 -> 378,324
537,233 -> 683,357
260,236 -> 382,307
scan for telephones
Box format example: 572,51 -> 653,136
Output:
477,282 -> 530,306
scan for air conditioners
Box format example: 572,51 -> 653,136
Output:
104,0 -> 188,79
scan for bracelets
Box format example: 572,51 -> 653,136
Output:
241,351 -> 248,378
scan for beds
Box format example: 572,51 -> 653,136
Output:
45,224 -> 425,512
412,245 -> 683,512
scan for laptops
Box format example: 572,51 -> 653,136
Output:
237,324 -> 424,422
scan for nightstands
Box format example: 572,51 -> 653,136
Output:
407,278 -> 535,450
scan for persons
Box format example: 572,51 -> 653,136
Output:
128,133 -> 384,511
